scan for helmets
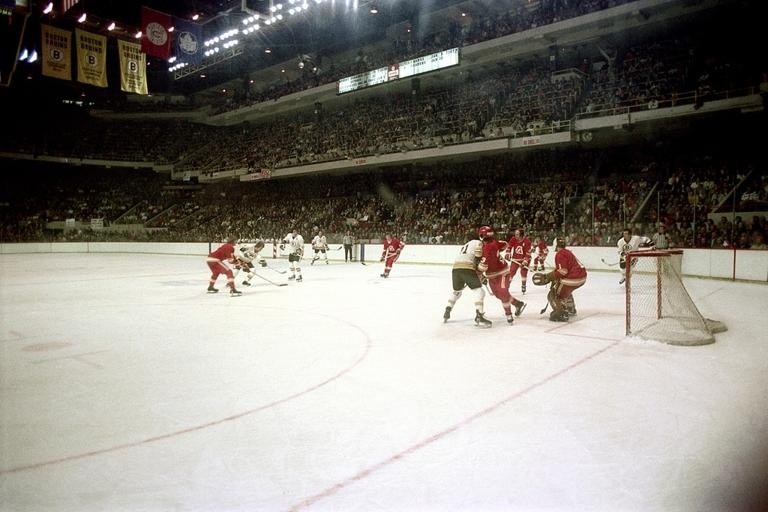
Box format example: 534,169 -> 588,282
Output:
553,237 -> 565,251
479,226 -> 494,241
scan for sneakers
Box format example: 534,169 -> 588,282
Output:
475,316 -> 492,324
522,285 -> 526,292
507,313 -> 513,322
208,275 -> 303,293
515,302 -> 523,315
444,310 -> 450,318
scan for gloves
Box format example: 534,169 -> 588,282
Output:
522,259 -> 528,266
247,262 -> 253,268
260,260 -> 266,267
243,268 -> 250,272
505,253 -> 511,261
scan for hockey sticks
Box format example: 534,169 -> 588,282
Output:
486,284 -> 495,296
266,264 -> 287,274
250,270 -> 288,287
602,258 -> 625,266
540,301 -> 549,314
361,253 -> 396,265
315,246 -> 342,250
